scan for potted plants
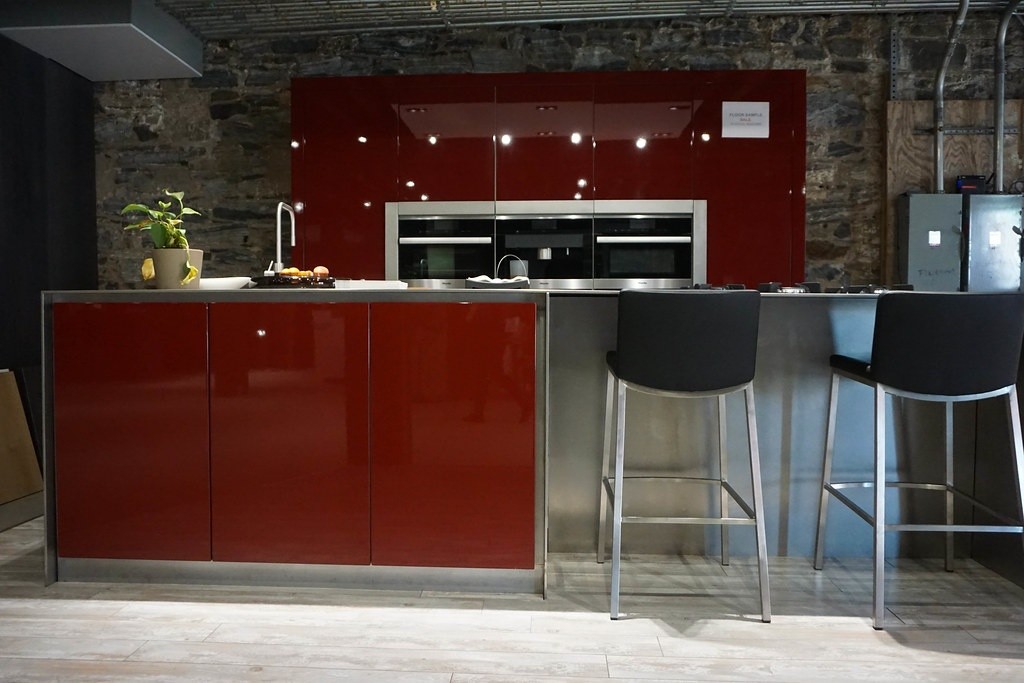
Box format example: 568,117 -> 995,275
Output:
123,190 -> 207,290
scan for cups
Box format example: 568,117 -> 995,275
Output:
510,260 -> 528,279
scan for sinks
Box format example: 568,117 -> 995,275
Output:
197,274 -> 410,292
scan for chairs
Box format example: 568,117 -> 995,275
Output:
814,289 -> 1024,644
599,289 -> 773,622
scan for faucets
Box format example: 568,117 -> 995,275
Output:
273,201 -> 296,273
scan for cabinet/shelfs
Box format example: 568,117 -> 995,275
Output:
283,71 -> 807,296
41,291 -> 551,600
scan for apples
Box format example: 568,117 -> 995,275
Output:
314,266 -> 329,276
